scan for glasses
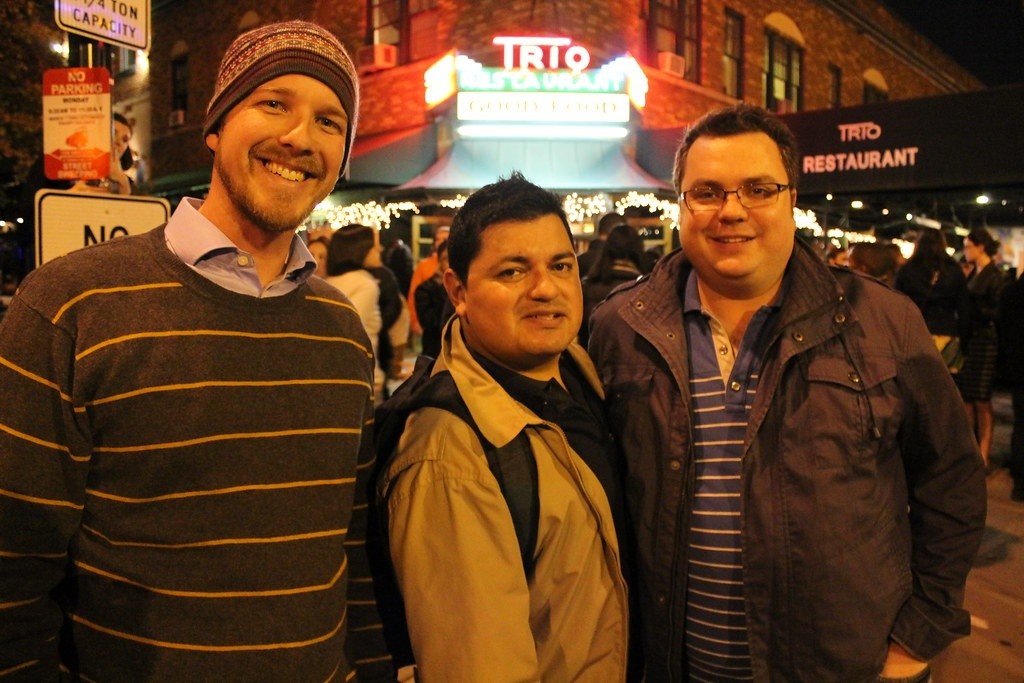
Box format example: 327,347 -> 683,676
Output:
679,182 -> 789,212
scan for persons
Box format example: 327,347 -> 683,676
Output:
18,112 -> 140,223
385,180 -> 642,683
1,20 -> 400,683
304,224 -> 454,402
824,228 -> 1024,504
577,212 -> 652,318
589,105 -> 987,683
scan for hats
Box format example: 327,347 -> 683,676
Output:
202,19 -> 359,181
